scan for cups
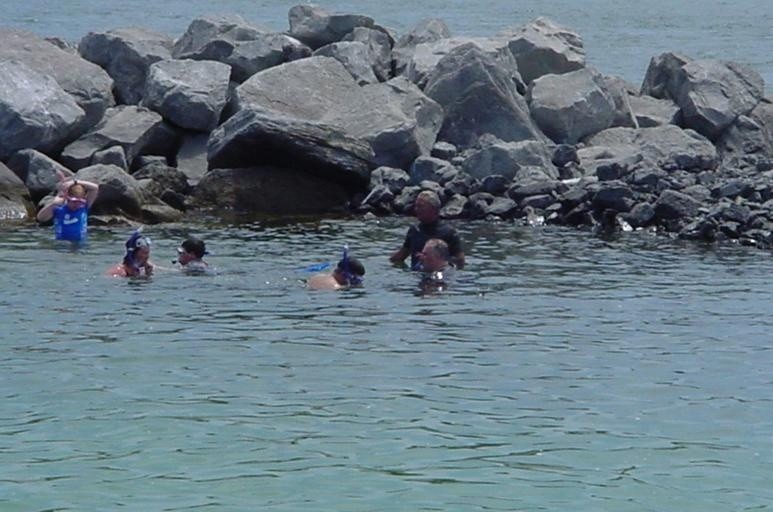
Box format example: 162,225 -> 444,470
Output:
65,197 -> 87,208
350,276 -> 364,285
136,236 -> 151,247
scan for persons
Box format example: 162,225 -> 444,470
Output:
420,238 -> 453,272
390,190 -> 465,270
178,238 -> 209,268
106,230 -> 178,277
308,258 -> 365,290
37,179 -> 99,241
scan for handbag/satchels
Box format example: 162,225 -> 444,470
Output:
73,179 -> 77,184
